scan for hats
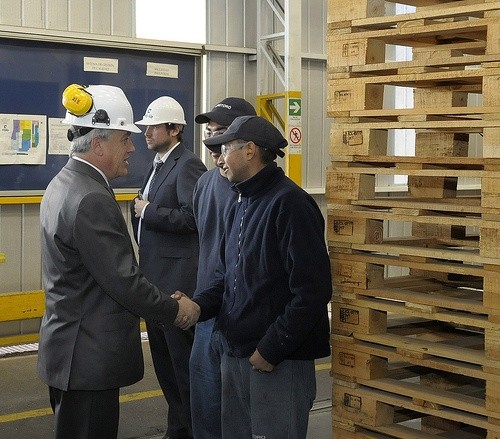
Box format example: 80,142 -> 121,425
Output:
195,97 -> 256,127
202,116 -> 288,158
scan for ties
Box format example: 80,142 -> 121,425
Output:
148,162 -> 162,195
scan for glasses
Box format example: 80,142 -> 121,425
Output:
203,130 -> 226,138
221,143 -> 247,157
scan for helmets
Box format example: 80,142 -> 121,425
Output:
134,96 -> 187,125
62,85 -> 142,133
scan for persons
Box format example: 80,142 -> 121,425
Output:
187,97 -> 257,439
130,96 -> 208,439
37,84 -> 200,439
173,116 -> 331,439
